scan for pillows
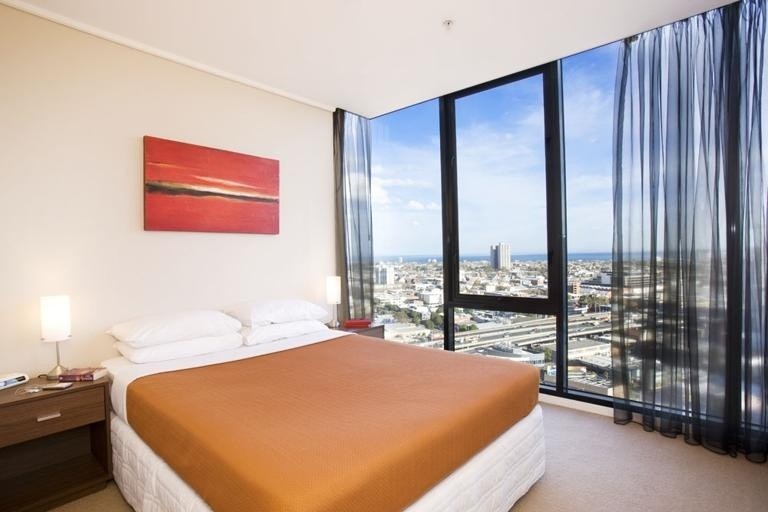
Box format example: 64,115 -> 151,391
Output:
106,297 -> 329,363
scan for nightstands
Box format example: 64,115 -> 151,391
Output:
0,368 -> 114,510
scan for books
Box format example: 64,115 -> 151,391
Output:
344,319 -> 371,327
58,367 -> 107,380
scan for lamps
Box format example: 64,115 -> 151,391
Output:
41,293 -> 73,380
324,278 -> 342,328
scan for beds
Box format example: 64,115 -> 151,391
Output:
96,325 -> 547,510
